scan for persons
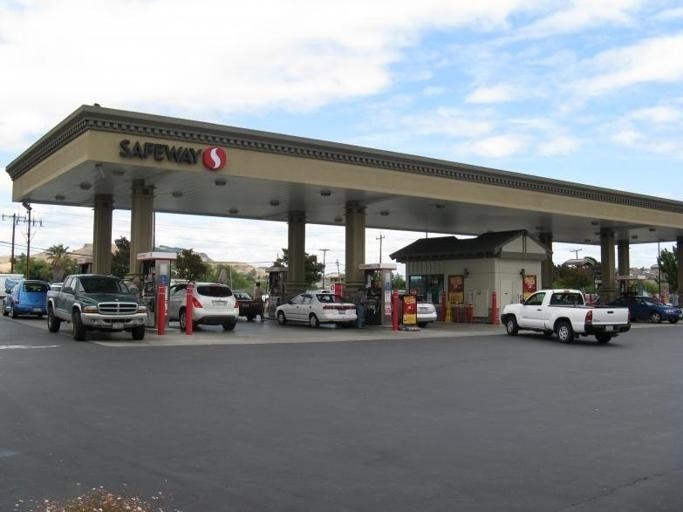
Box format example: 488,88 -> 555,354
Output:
253,281 -> 267,321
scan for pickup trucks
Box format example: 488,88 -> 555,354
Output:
501,289 -> 631,343
44,273 -> 151,341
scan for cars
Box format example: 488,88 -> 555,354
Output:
169,281 -> 239,332
390,291 -> 438,328
234,292 -> 264,322
609,296 -> 683,324
274,292 -> 358,328
2,279 -> 52,320
